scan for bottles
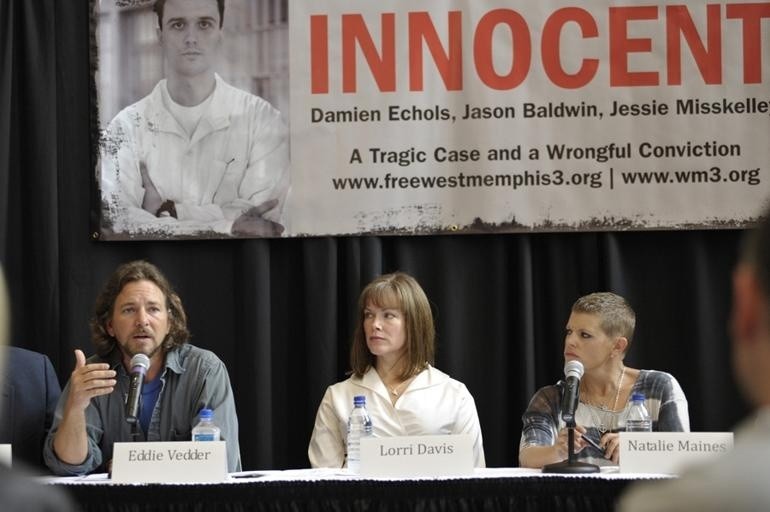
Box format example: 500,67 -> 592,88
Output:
625,392 -> 654,434
190,408 -> 222,440
345,396 -> 373,469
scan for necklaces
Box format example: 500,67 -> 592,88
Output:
387,382 -> 403,395
584,367 -> 625,450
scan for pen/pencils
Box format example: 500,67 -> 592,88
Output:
581,433 -> 606,454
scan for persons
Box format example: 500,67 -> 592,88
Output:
518,292 -> 690,467
95,0 -> 290,238
617,214 -> 770,512
42,261 -> 242,476
0,345 -> 62,474
308,271 -> 486,469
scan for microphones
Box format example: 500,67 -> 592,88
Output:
125,353 -> 151,423
562,360 -> 585,421
